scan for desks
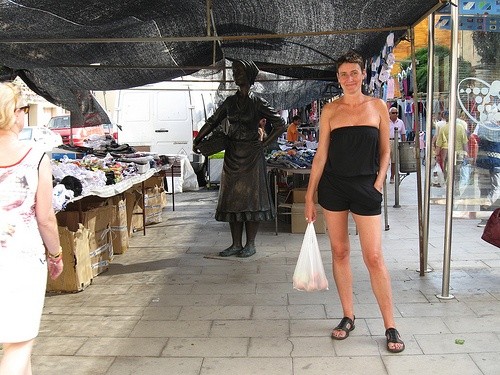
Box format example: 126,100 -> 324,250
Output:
266,166 -> 311,236
55,154 -> 186,236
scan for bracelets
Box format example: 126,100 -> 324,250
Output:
48,245 -> 63,265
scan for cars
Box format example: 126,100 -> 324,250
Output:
19,125 -> 63,153
44,111 -> 118,149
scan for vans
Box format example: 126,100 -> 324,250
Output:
111,72 -> 227,173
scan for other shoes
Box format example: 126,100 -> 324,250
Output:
477,220 -> 486,226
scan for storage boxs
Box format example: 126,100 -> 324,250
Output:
47,177 -> 165,293
284,188 -> 318,203
279,203 -> 326,234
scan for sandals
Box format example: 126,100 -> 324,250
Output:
384,328 -> 405,353
332,315 -> 355,340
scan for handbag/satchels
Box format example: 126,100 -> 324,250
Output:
293,222 -> 329,292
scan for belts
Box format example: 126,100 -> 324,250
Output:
389,138 -> 394,140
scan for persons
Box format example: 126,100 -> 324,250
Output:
258,118 -> 267,141
287,115 -> 306,144
386,107 -> 406,184
431,94 -> 500,227
192,59 -> 285,258
0,82 -> 63,375
306,52 -> 405,353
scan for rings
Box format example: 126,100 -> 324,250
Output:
305,218 -> 309,221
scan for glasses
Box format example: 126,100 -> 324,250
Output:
15,106 -> 30,115
391,112 -> 398,114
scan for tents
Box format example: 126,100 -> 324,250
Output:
1,0 -> 461,301
